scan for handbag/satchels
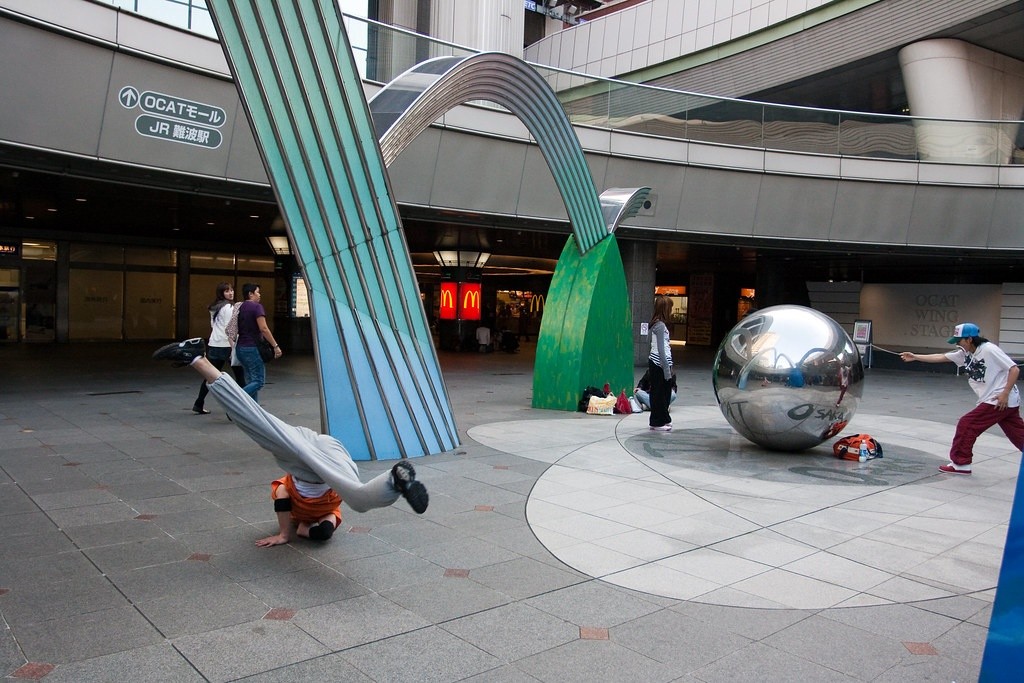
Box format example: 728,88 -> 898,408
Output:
231,334 -> 243,366
258,340 -> 274,362
579,385 -> 643,415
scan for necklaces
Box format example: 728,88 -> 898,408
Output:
964,344 -> 980,370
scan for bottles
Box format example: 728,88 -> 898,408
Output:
859,440 -> 867,463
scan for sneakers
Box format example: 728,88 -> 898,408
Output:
649,423 -> 673,430
392,461 -> 429,514
836,403 -> 840,407
153,337 -> 207,366
939,462 -> 971,475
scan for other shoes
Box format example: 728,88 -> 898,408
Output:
193,407 -> 211,414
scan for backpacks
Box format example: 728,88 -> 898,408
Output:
833,434 -> 883,461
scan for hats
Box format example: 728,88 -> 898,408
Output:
947,323 -> 980,344
841,360 -> 845,364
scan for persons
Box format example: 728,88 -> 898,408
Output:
646,295 -> 675,431
223,282 -> 282,422
192,282 -> 238,414
226,297 -> 247,402
423,286 -> 552,362
898,323 -> 1024,478
146,337 -> 431,550
633,356 -> 678,414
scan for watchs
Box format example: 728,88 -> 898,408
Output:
274,343 -> 279,349
